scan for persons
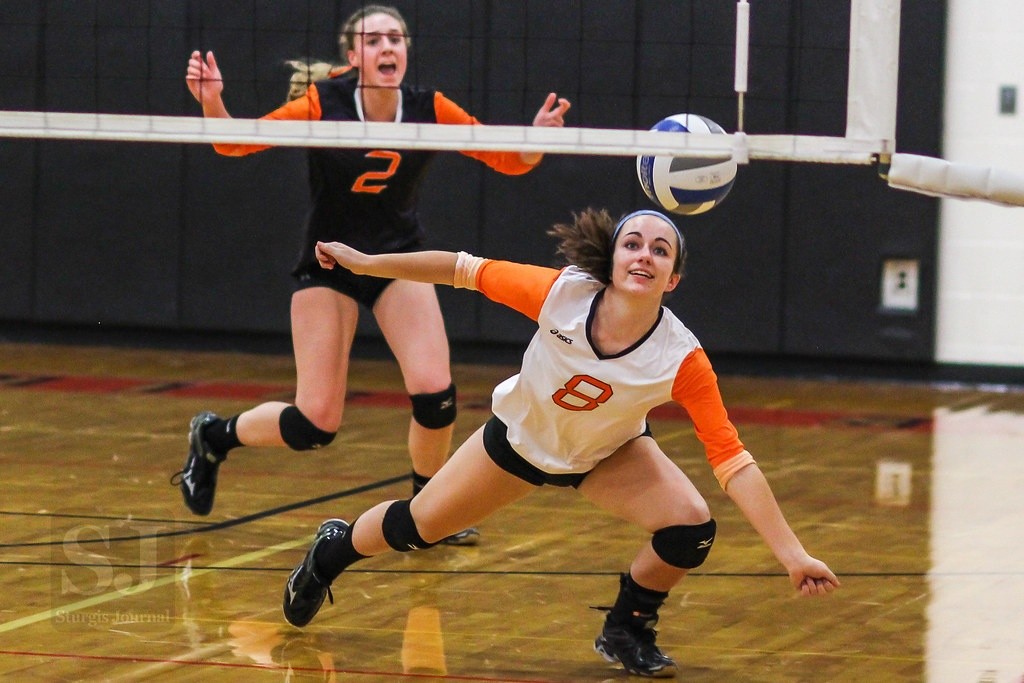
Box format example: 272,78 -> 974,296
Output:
281,210 -> 841,677
179,537 -> 479,682
181,6 -> 571,543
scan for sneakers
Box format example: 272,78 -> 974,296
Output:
589,606 -> 678,678
284,518 -> 350,628
443,527 -> 480,544
170,412 -> 227,517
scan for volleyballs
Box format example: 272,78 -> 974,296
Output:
636,113 -> 739,217
